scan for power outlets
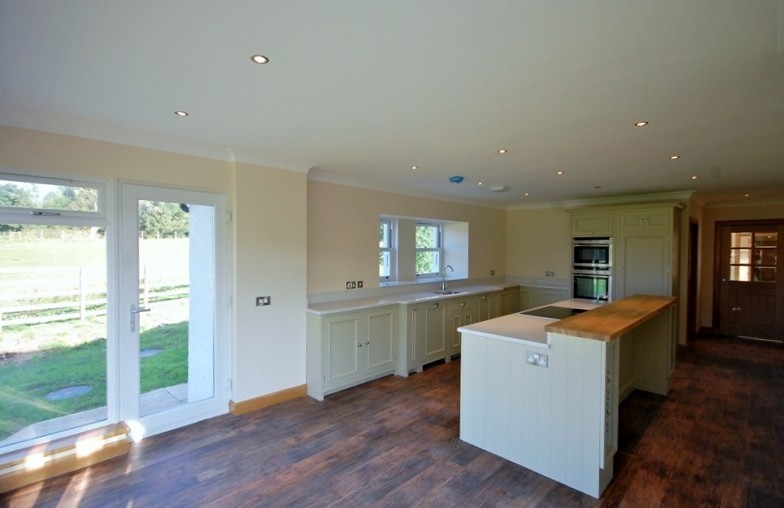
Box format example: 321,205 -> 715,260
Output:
345,281 -> 356,289
545,270 -> 550,276
357,280 -> 364,289
489,269 -> 496,277
525,351 -> 549,369
550,270 -> 555,277
256,296 -> 270,306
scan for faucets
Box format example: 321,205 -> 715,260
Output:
440,265 -> 454,291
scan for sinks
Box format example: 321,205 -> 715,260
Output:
431,290 -> 464,296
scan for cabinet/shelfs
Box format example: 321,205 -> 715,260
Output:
306,303 -> 399,401
615,201 -> 687,300
568,204 -> 617,239
395,287 -> 519,379
